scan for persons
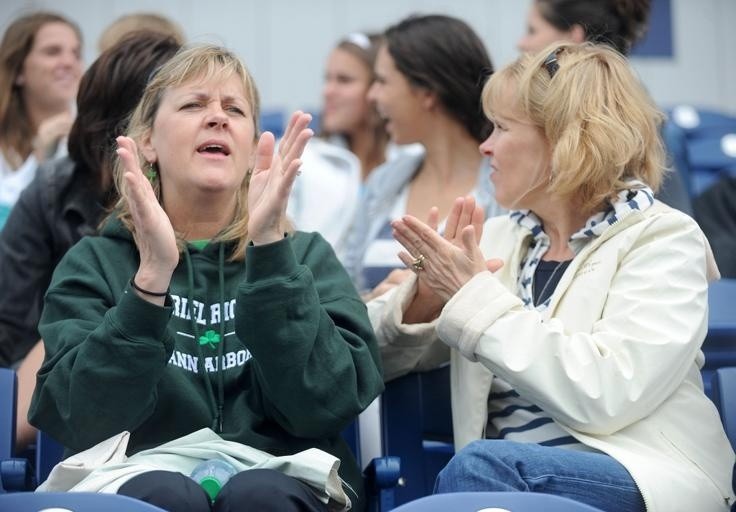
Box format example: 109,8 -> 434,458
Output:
514,1 -> 696,218
25,39 -> 393,511
691,177 -> 735,285
316,11 -> 508,476
360,37 -> 736,512
1,10 -> 85,238
95,9 -> 188,57
0,28 -> 188,461
261,31 -> 424,257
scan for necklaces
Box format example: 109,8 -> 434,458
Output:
532,238 -> 576,308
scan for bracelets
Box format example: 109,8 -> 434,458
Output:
130,275 -> 172,297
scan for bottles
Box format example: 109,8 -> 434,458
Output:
188,458 -> 234,502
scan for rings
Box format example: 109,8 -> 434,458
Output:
411,253 -> 425,271
